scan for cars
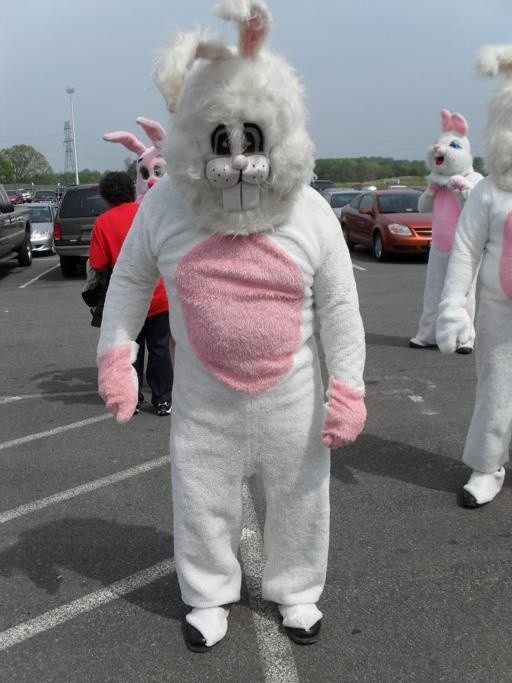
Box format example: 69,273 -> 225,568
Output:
321,188 -> 363,219
339,188 -> 432,261
310,179 -> 427,191
14,203 -> 56,254
6,185 -> 67,206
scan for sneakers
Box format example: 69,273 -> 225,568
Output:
156,402 -> 171,415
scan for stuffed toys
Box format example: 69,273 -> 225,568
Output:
101,114 -> 177,368
93,0 -> 368,653
406,107 -> 485,356
434,43 -> 511,508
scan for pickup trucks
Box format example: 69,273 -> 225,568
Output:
0,183 -> 34,268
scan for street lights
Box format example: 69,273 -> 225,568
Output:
67,86 -> 82,186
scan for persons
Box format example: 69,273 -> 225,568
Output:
86,168 -> 173,420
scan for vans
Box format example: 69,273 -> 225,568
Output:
53,182 -> 112,277
